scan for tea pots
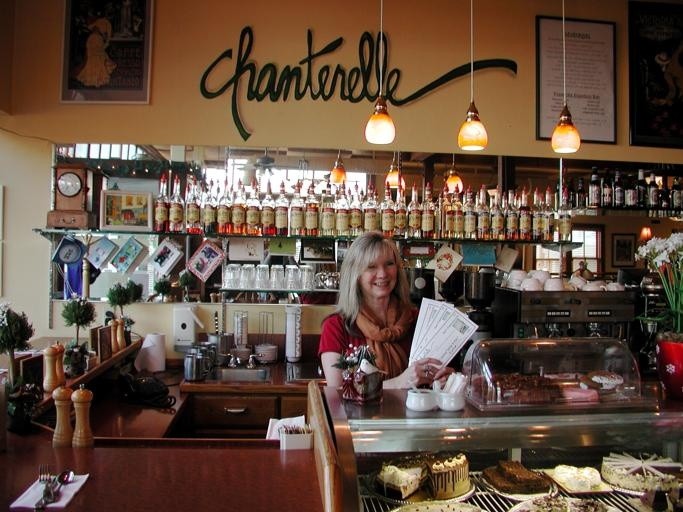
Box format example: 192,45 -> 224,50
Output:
319,273 -> 340,289
184,355 -> 211,382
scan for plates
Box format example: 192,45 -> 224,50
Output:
256,346 -> 279,364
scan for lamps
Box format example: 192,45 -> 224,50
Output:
363,0 -> 398,147
544,0 -> 584,158
327,149 -> 347,186
453,0 -> 491,154
638,226 -> 654,270
384,150 -> 407,190
442,152 -> 463,194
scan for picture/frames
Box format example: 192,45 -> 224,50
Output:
54,1 -> 159,109
626,3 -> 683,151
609,232 -> 639,268
531,15 -> 620,148
97,188 -> 155,233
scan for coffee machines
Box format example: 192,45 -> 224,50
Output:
493,293 -> 638,375
411,269 -> 497,363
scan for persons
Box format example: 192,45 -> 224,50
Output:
317,231 -> 456,390
201,247 -> 217,264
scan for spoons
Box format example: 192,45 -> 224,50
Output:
52,469 -> 75,500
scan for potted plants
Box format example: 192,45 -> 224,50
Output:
103,283 -> 132,347
57,297 -> 96,379
0,301 -> 37,436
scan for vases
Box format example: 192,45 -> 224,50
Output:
656,332 -> 682,388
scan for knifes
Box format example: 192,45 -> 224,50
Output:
34,471 -> 64,511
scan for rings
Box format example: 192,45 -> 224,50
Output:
423,364 -> 432,372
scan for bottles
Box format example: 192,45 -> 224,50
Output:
155,174 -> 572,244
575,164 -> 683,212
108,318 -> 127,352
41,341 -> 95,447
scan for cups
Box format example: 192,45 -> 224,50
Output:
285,305 -> 301,362
221,263 -> 315,291
503,269 -> 627,292
402,389 -> 469,413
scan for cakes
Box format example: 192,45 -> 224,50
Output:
374,450 -> 683,512
470,370 -> 625,405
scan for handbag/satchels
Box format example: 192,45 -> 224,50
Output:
116,372 -> 168,403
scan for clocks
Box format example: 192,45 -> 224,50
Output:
44,162 -> 96,231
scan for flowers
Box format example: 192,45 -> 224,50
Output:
633,233 -> 683,335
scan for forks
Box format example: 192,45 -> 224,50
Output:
37,464 -> 54,503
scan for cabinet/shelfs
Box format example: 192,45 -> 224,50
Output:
183,378 -> 309,437
322,374 -> 682,510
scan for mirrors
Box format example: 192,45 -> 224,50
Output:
52,143 -> 556,241
50,233 -> 338,307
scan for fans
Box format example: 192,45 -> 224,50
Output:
231,148 -> 290,177
